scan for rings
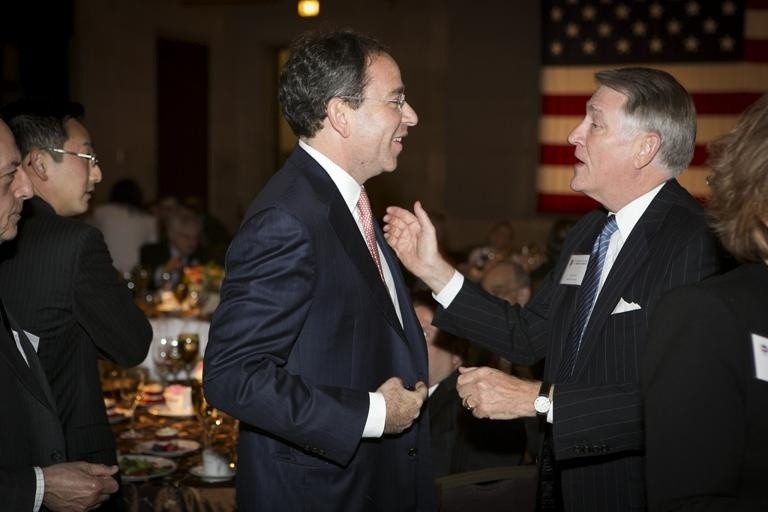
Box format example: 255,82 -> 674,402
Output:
463,394 -> 473,412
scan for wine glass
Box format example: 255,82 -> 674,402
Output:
192,381 -> 224,449
150,324 -> 177,386
117,371 -> 143,440
177,316 -> 200,383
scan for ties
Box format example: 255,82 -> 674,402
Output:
356,190 -> 384,285
558,215 -> 618,379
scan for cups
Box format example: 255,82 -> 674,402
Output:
201,450 -> 227,476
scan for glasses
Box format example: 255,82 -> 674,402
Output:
42,147 -> 98,168
341,94 -> 405,110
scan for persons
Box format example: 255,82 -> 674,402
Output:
0,119 -> 121,512
86,178 -> 231,293
407,286 -> 526,486
2,98 -> 152,512
383,66 -> 720,511
642,93 -> 768,511
204,25 -> 436,510
484,260 -> 532,308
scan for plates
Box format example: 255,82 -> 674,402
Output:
118,455 -> 177,481
192,468 -> 236,482
138,437 -> 201,455
149,406 -> 196,418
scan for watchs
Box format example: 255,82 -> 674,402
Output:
532,379 -> 553,432
